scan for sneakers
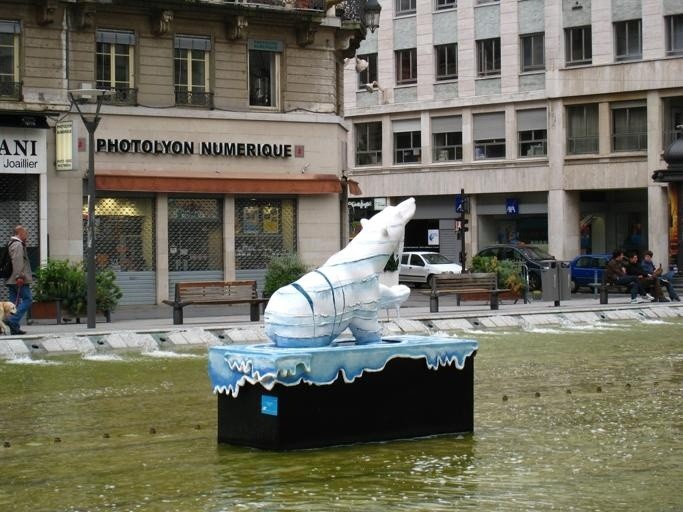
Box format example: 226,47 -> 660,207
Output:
631,293 -> 654,303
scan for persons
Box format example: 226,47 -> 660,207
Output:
1,225 -> 34,334
607,249 -> 654,303
622,252 -> 669,302
641,250 -> 681,302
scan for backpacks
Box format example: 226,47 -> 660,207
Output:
0,239 -> 20,279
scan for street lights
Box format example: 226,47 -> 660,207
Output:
69,88 -> 115,328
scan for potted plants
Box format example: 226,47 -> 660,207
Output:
27,255 -> 123,325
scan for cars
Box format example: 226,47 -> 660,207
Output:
476,244 -> 566,290
399,251 -> 467,288
570,255 -> 612,294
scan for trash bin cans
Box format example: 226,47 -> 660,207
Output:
540,260 -> 572,302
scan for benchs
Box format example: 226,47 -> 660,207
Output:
588,271 -> 668,304
417,272 -> 512,312
161,280 -> 271,325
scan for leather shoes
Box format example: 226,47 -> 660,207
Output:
660,278 -> 670,287
11,326 -> 27,335
655,293 -> 680,303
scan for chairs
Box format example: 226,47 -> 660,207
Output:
526,146 -> 544,155
475,147 -> 485,160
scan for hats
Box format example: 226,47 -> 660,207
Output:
644,251 -> 653,257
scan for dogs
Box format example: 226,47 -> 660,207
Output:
0,301 -> 18,335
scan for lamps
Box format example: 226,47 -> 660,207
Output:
364,81 -> 387,104
354,55 -> 369,73
360,0 -> 382,33
